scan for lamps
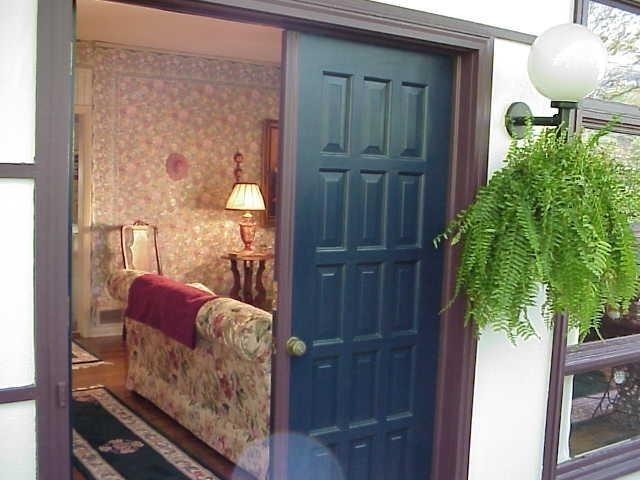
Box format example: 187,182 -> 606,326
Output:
507,19 -> 609,140
224,181 -> 266,257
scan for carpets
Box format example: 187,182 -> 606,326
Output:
70,339 -> 229,480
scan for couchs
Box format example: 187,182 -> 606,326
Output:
105,270 -> 272,480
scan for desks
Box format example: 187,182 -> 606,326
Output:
219,251 -> 273,304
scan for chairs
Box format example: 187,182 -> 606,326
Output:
120,220 -> 163,278
